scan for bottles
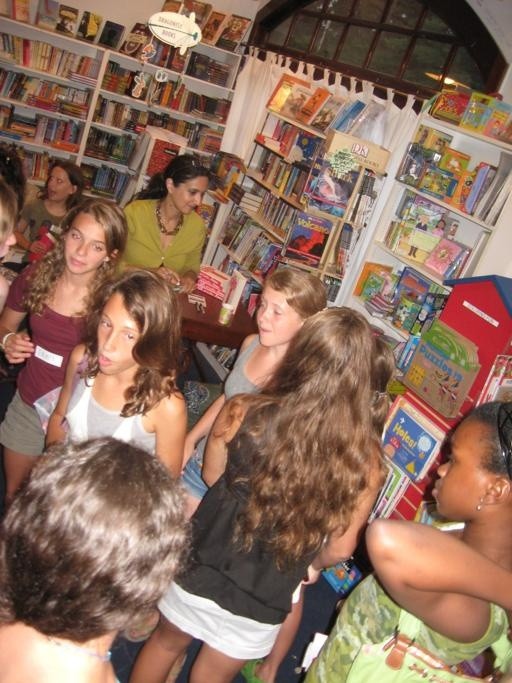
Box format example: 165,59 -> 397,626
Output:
218,303 -> 234,325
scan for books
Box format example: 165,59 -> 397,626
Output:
198,75 -> 376,370
354,126 -> 497,528
0,0 -> 251,204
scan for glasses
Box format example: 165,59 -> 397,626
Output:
170,160 -> 210,176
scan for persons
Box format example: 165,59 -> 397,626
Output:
304,401 -> 512,683
0,435 -> 192,683
129,265 -> 396,683
0,154 -> 210,522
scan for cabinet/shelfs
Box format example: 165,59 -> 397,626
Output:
331,114 -> 511,367
0,15 -> 242,214
205,107 -> 389,312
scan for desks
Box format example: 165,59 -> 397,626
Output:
175,277 -> 255,379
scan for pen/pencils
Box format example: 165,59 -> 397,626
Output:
196,301 -> 199,311
199,302 -> 204,314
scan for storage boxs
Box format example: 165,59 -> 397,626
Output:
322,128 -> 389,176
